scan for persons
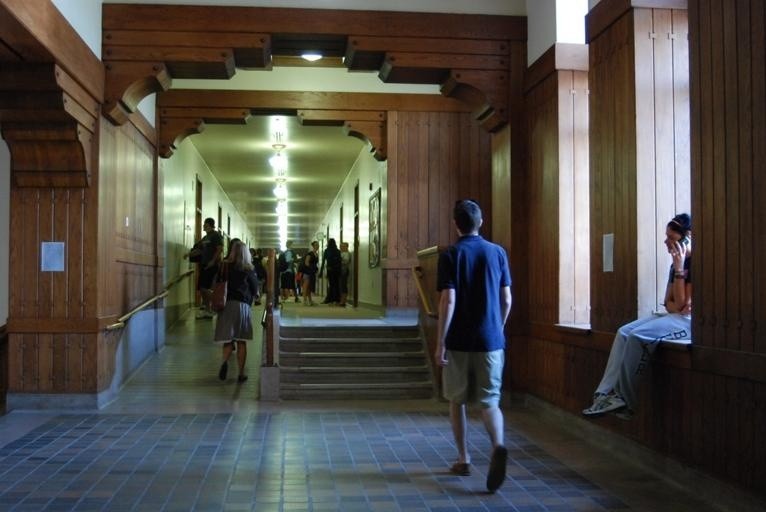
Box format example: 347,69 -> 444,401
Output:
433,197 -> 513,494
580,212 -> 692,421
182,217 -> 351,383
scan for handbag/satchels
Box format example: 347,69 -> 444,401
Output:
210,260 -> 229,311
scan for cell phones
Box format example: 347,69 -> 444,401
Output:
675,236 -> 689,251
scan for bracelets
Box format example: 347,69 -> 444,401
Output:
674,271 -> 688,279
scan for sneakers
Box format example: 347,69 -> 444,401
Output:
237,374 -> 249,382
581,394 -> 626,415
218,362 -> 228,381
607,410 -> 633,421
486,444 -> 509,492
197,306 -> 211,321
282,298 -> 347,308
452,463 -> 473,476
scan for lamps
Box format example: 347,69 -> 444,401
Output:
268,114 -> 289,253
301,54 -> 322,62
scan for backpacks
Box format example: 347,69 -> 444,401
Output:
297,252 -> 312,274
276,251 -> 288,271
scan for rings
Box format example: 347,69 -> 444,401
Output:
673,252 -> 676,257
676,252 -> 679,254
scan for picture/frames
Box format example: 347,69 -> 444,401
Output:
368,187 -> 381,268
